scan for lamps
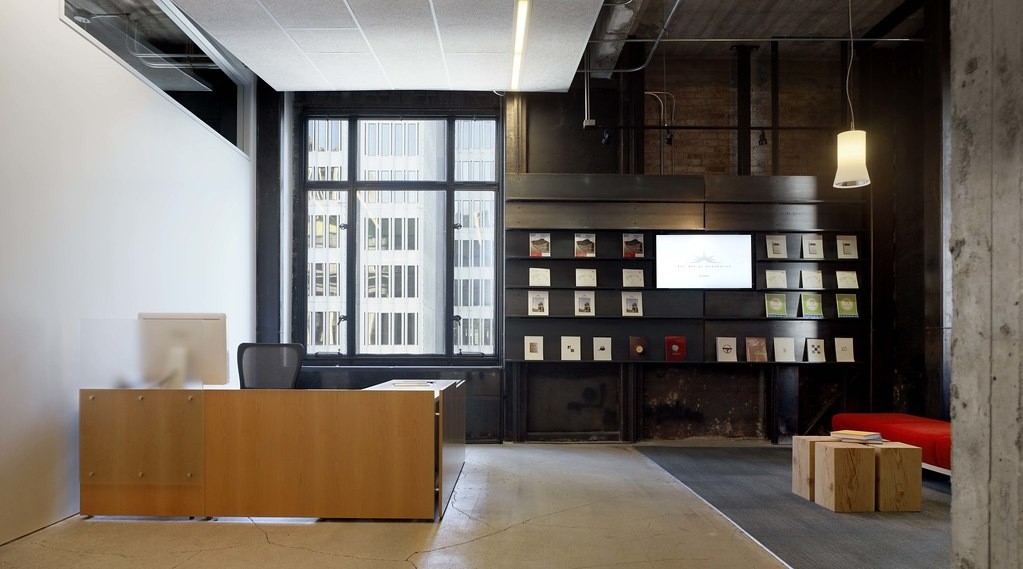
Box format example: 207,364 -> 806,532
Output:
833,0 -> 871,189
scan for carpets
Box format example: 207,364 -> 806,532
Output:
632,445 -> 952,569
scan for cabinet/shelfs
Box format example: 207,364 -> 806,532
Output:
503,174 -> 872,447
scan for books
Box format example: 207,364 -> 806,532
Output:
842,440 -> 882,444
831,430 -> 882,440
523,233 -> 858,363
393,381 -> 431,386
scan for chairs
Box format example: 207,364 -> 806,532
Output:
237,343 -> 305,389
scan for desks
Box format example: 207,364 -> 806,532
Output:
791,436 -> 922,513
301,366 -> 503,444
79,379 -> 466,520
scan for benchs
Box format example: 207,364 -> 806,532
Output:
832,413 -> 951,496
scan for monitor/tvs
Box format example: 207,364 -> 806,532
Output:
653,230 -> 755,290
